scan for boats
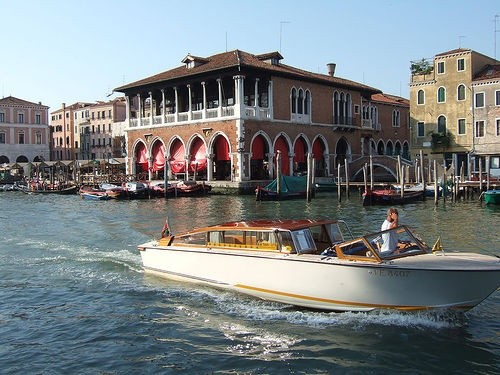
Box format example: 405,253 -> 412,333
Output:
255,175 -> 321,201
137,217 -> 500,317
21,177 -> 77,195
362,189 -> 424,205
483,189 -> 500,206
79,180 -> 212,201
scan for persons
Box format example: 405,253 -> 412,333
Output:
381,207 -> 410,251
